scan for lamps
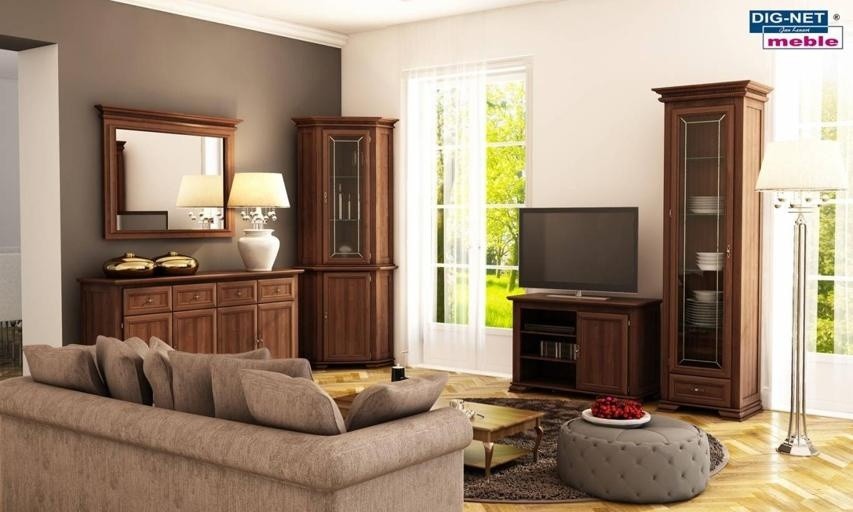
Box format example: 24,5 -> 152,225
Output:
754,140 -> 848,456
227,172 -> 290,272
175,175 -> 224,229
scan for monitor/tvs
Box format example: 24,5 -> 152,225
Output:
116,211 -> 168,231
518,206 -> 640,301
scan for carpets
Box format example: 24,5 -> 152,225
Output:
458,398 -> 730,503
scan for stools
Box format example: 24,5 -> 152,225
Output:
558,417 -> 711,504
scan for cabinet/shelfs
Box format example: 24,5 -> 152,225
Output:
79,283 -> 218,355
292,266 -> 400,369
290,116 -> 399,266
218,277 -> 295,359
651,79 -> 775,421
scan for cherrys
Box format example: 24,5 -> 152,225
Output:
590,396 -> 644,420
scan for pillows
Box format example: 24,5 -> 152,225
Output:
94,336 -> 143,405
166,345 -> 274,414
23,343 -> 97,395
208,356 -> 309,422
239,366 -> 347,436
142,335 -> 179,409
345,372 -> 449,428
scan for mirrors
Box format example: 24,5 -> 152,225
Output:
95,102 -> 244,240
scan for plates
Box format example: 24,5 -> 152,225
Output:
582,408 -> 651,428
695,251 -> 724,271
687,195 -> 726,215
684,289 -> 722,329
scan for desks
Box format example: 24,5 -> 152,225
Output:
507,293 -> 663,405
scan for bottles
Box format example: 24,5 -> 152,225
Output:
345,193 -> 351,220
334,184 -> 344,221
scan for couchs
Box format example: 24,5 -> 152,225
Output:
0,376 -> 474,512
